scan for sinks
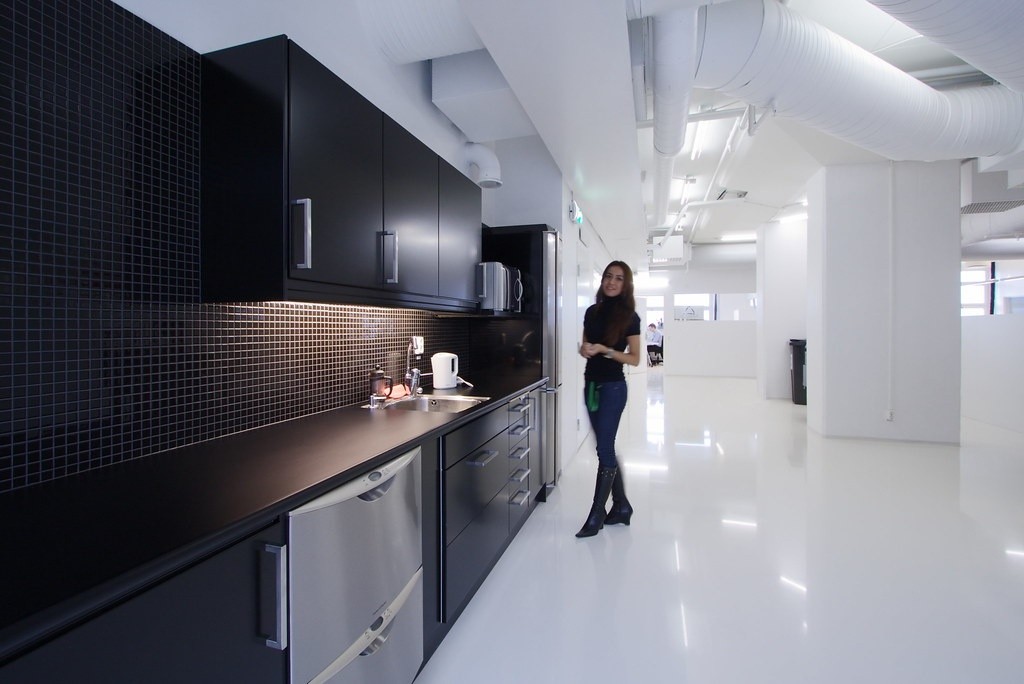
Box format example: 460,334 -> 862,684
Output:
361,395 -> 492,413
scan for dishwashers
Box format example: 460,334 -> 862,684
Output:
285,449 -> 424,684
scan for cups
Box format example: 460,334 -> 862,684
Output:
370,365 -> 392,399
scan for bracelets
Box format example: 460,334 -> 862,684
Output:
604,348 -> 614,359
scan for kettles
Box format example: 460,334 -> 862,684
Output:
430,352 -> 459,389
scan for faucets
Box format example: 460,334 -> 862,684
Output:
404,336 -> 434,398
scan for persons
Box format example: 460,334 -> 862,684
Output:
647,323 -> 662,352
575,260 -> 641,537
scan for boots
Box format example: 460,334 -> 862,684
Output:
575,465 -> 617,537
604,466 -> 633,526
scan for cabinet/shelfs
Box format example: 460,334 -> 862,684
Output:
358,375 -> 549,631
201,34 -> 484,314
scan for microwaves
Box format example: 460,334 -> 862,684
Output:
483,262 -> 521,313
